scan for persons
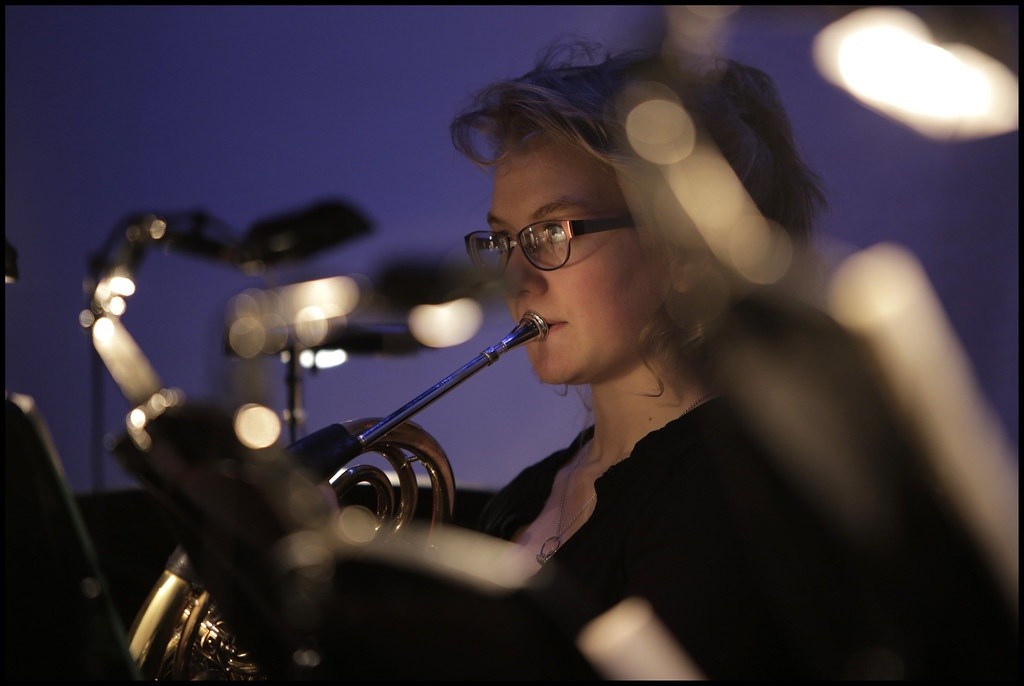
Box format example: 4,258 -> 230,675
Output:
457,47 -> 822,686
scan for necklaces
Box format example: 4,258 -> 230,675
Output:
534,440 -> 595,564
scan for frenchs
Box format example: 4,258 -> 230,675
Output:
124,310 -> 548,686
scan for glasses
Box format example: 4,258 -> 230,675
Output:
464,215 -> 633,280
